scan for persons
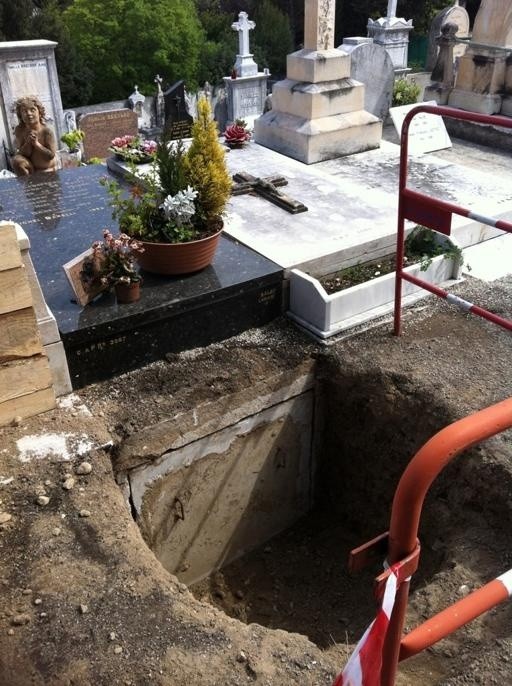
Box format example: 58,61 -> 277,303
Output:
18,170 -> 63,232
7,95 -> 57,177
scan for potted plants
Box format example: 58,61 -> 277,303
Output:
102,89 -> 233,274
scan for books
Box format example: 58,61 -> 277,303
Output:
61,239 -> 115,309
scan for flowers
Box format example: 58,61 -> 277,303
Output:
86,225 -> 146,284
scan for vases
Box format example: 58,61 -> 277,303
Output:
112,278 -> 139,304
